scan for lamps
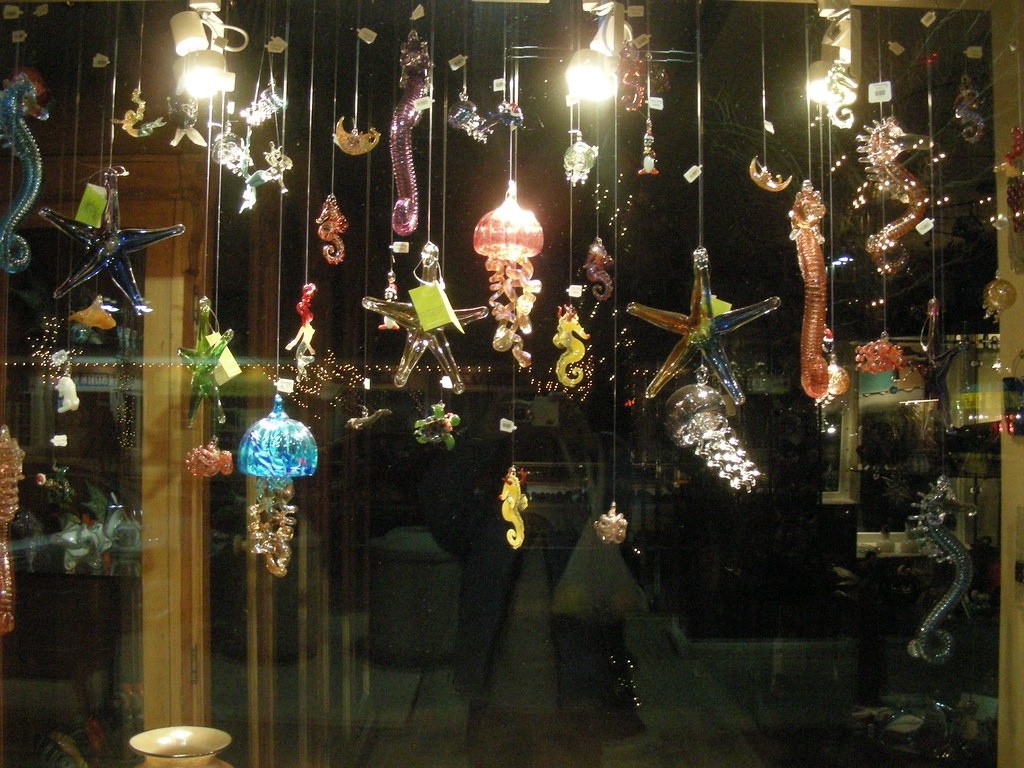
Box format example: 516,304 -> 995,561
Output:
169,0 -> 866,483
